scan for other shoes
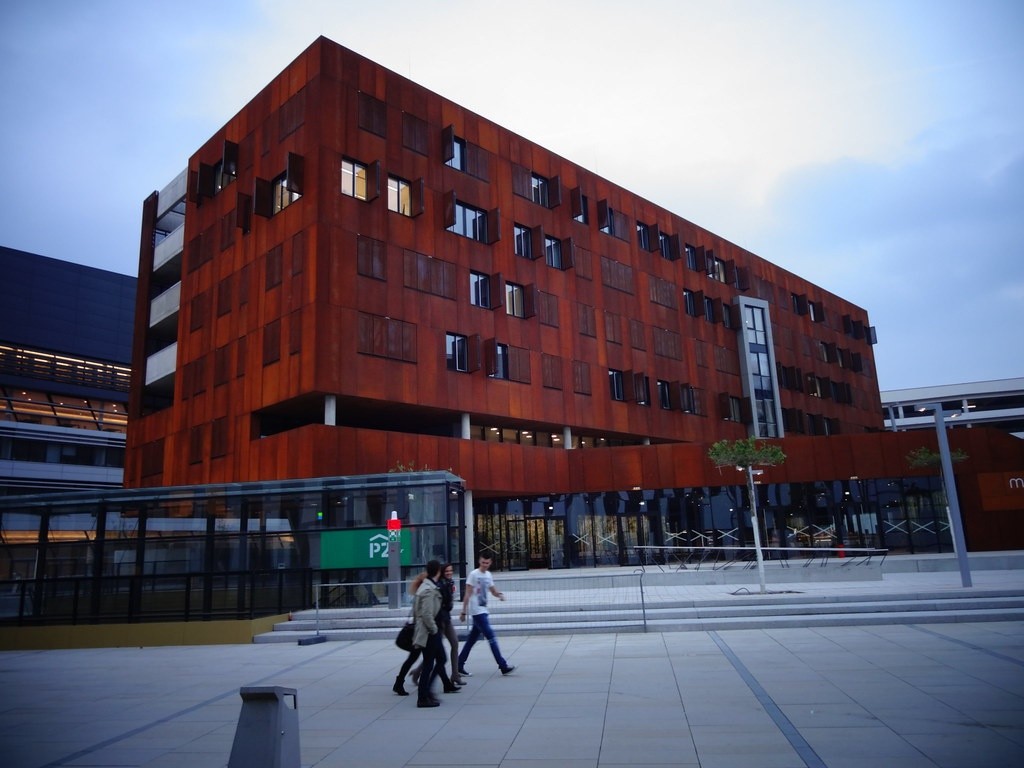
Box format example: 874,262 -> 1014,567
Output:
458,670 -> 471,676
417,698 -> 440,707
501,665 -> 516,676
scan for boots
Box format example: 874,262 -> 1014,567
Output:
393,676 -> 409,696
443,679 -> 461,693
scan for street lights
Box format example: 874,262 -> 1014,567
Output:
915,403 -> 973,587
735,464 -> 767,594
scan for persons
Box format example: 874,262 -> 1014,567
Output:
409,562 -> 468,685
459,549 -> 518,676
412,560 -> 443,709
392,570 -> 462,695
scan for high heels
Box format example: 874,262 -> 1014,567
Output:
451,675 -> 466,685
410,669 -> 419,685
